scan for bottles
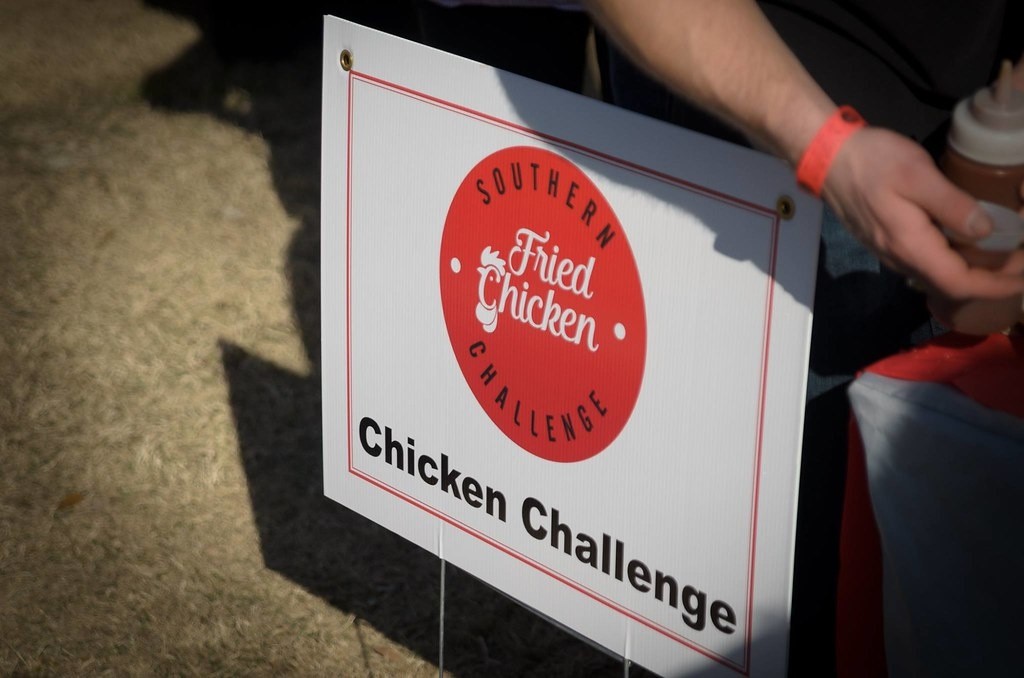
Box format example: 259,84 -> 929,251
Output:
938,62 -> 1023,337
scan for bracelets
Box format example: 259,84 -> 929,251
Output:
797,105 -> 867,197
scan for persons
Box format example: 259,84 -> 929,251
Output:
575,0 -> 1022,677
